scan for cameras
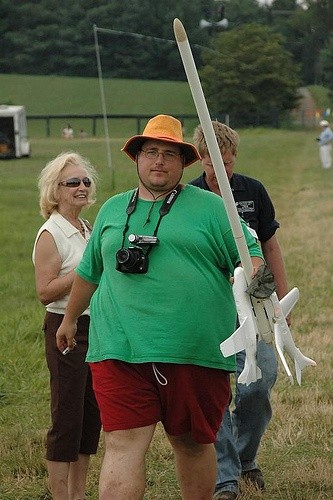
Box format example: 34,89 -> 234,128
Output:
115,246 -> 149,274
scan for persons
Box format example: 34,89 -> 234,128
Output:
187,121 -> 292,500
32,153 -> 102,500
319,120 -> 333,169
64,124 -> 73,139
56,114 -> 267,500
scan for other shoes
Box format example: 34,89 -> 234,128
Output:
214,490 -> 238,500
241,469 -> 264,491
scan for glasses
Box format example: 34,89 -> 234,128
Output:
58,177 -> 91,188
139,149 -> 183,161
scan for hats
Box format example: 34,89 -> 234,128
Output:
121,115 -> 202,167
319,120 -> 329,127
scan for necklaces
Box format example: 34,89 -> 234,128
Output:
76,224 -> 85,235
